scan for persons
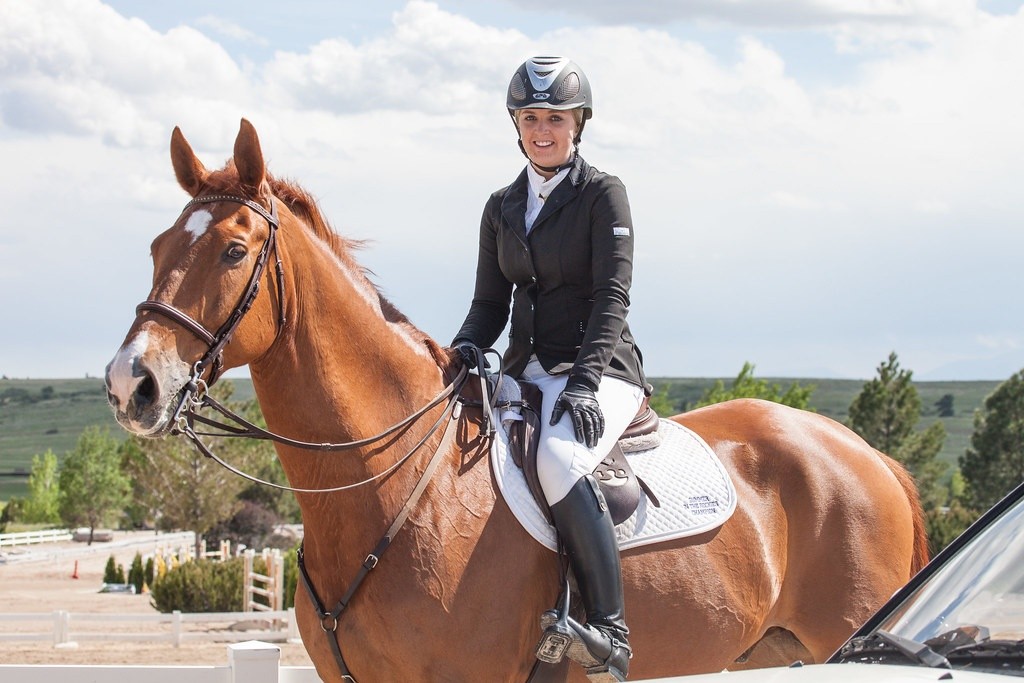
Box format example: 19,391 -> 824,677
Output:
449,53 -> 651,683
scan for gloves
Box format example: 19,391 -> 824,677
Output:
549,375 -> 605,449
452,340 -> 491,370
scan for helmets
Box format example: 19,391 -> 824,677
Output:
506,55 -> 593,120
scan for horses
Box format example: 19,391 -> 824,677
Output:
104,118 -> 930,683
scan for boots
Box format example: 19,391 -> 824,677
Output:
539,473 -> 631,683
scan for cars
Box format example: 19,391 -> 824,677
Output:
788,479 -> 1024,680
